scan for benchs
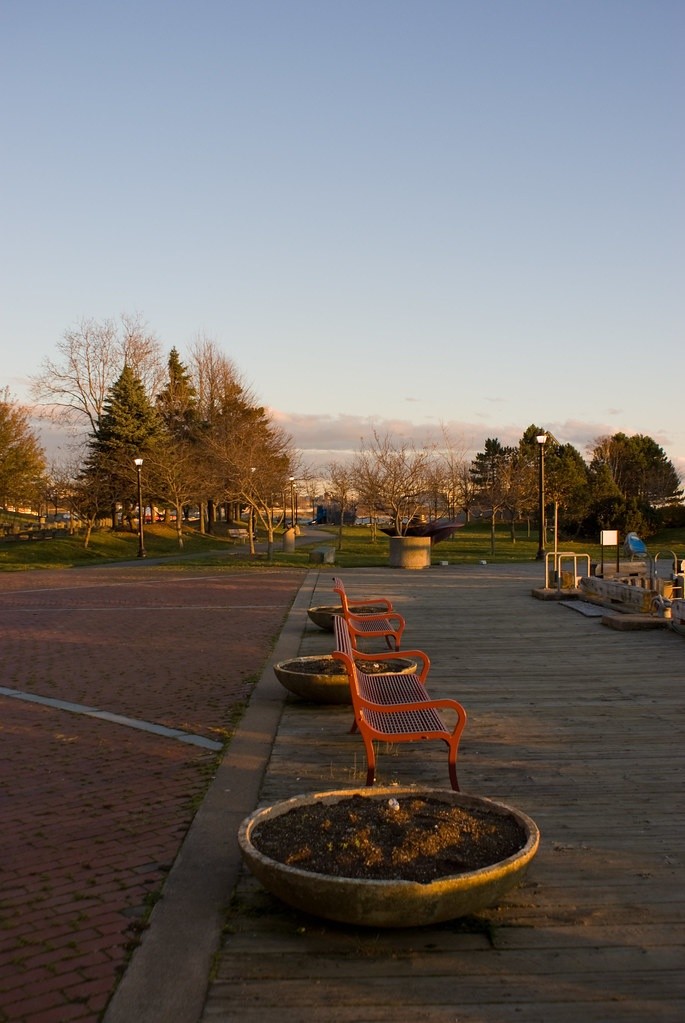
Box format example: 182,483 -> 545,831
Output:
332,614 -> 467,793
228,528 -> 256,545
331,576 -> 406,651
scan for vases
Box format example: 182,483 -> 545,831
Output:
308,605 -> 397,632
274,654 -> 418,704
238,783 -> 541,928
